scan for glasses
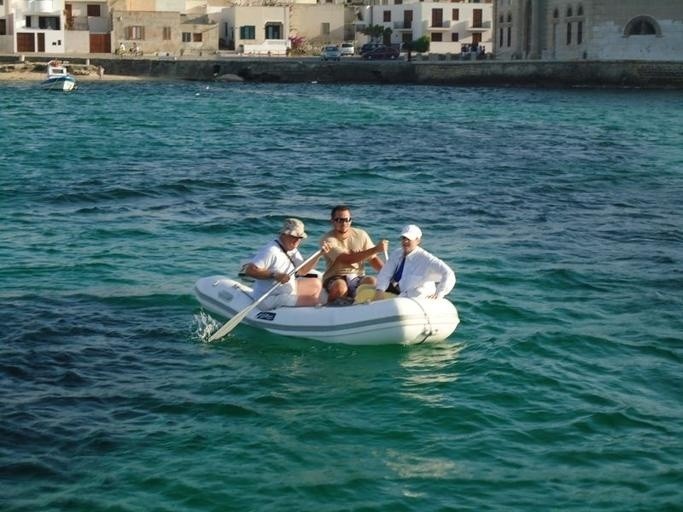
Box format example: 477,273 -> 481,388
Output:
333,217 -> 352,223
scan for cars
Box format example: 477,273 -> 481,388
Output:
362,47 -> 399,59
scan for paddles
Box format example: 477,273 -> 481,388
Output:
208,247 -> 325,343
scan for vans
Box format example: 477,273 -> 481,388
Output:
320,43 -> 356,62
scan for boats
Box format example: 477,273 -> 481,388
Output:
41,65 -> 77,92
192,275 -> 460,344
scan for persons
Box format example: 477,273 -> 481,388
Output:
581,49 -> 588,59
245,218 -> 332,311
116,42 -> 138,57
354,224 -> 456,306
320,205 -> 390,303
458,43 -> 485,61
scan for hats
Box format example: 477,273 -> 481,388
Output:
279,218 -> 307,238
400,224 -> 422,241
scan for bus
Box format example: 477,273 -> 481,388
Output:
359,44 -> 401,53
